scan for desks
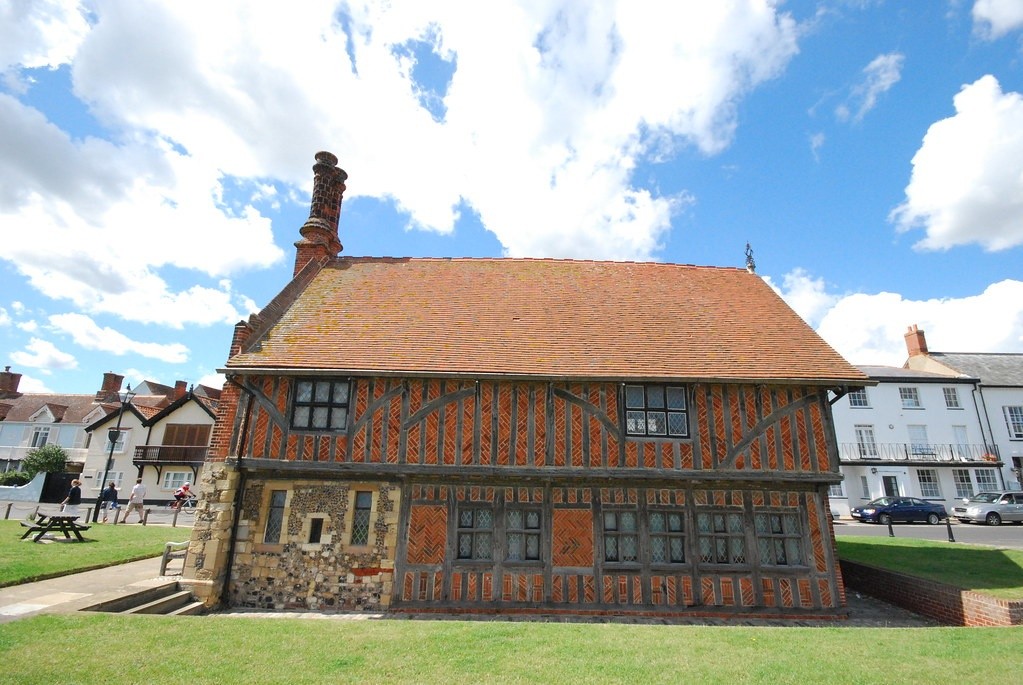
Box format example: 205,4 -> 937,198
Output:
20,512 -> 85,542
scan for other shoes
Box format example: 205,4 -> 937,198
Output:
118,520 -> 125,523
138,519 -> 143,523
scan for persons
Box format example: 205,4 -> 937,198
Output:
61,479 -> 81,520
98,482 -> 117,523
119,478 -> 146,523
171,482 -> 196,510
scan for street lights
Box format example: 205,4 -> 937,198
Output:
92,382 -> 137,524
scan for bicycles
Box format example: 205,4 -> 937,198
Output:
165,495 -> 198,514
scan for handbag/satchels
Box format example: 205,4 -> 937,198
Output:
100,502 -> 107,509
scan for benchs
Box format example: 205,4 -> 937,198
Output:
159,539 -> 189,576
19,520 -> 92,531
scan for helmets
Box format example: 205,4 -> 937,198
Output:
185,482 -> 190,486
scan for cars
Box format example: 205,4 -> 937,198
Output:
951,490 -> 1023,526
850,495 -> 947,525
